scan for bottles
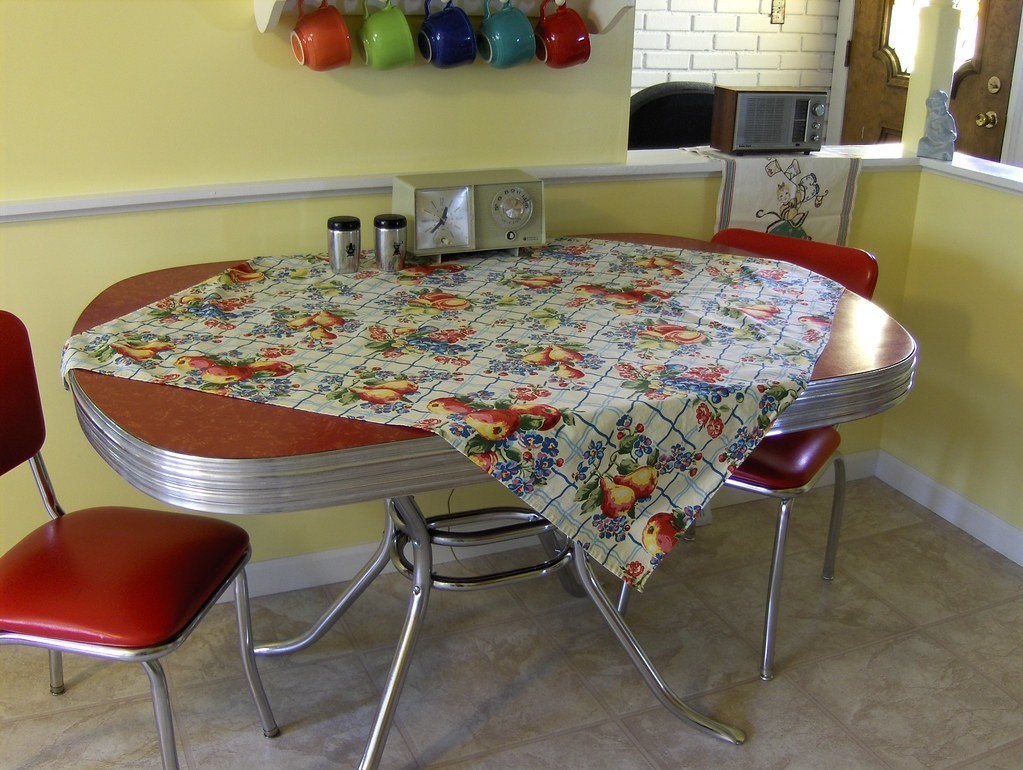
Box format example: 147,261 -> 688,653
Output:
328,216 -> 360,274
374,214 -> 407,272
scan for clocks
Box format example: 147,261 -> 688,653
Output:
389,167 -> 547,263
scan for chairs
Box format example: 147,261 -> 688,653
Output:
627,78 -> 717,148
0,305 -> 280,770
606,229 -> 882,682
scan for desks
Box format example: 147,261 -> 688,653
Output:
62,231 -> 916,770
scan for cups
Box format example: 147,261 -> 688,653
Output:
478,0 -> 539,68
357,0 -> 416,69
534,0 -> 591,69
418,0 -> 476,69
290,0 -> 351,71
